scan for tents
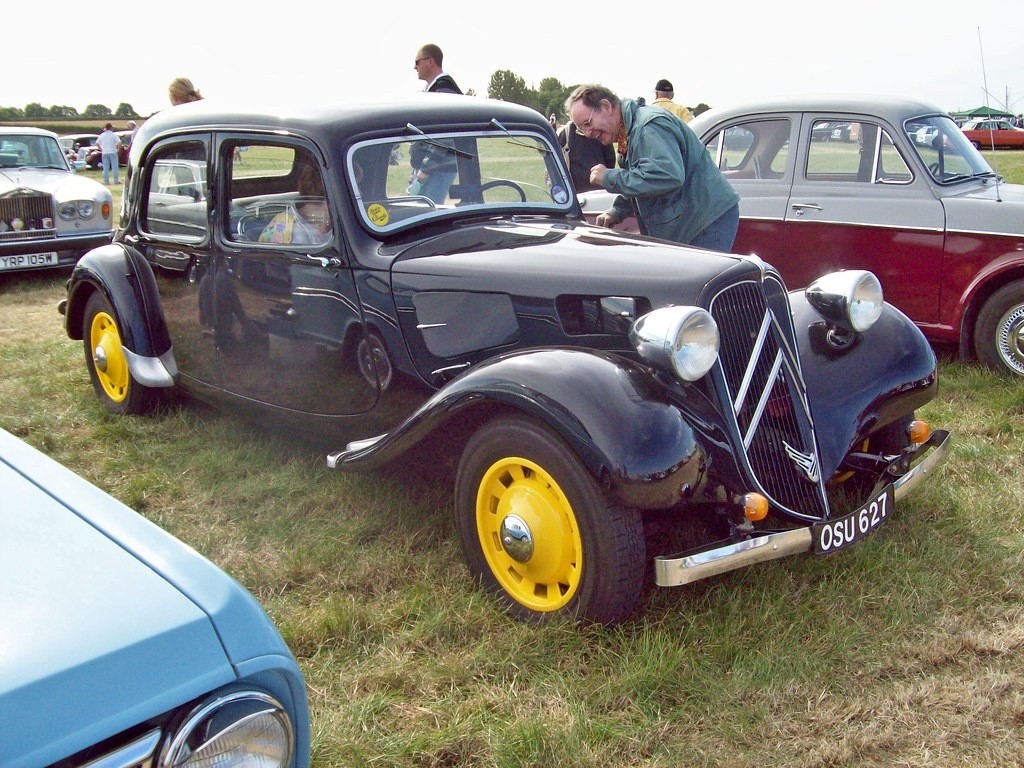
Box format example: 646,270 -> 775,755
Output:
955,106 -> 1014,119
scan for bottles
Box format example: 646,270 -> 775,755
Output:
406,179 -> 427,195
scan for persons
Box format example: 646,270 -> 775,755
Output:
65,143 -> 85,161
564,83 -> 742,253
548,118 -> 617,193
687,104 -> 710,117
95,123 -> 122,184
1000,114 -> 1024,129
168,77 -> 241,196
652,79 -> 692,126
126,121 -> 139,162
198,257 -> 258,345
259,156 -> 364,246
409,44 -> 463,205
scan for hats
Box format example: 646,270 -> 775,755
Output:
655,79 -> 673,91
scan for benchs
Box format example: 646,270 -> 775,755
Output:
146,189 -> 300,244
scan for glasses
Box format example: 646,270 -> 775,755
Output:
574,107 -> 596,136
415,57 -> 430,65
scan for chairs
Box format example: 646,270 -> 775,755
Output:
237,209 -> 285,244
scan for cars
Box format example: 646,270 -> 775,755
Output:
56,86 -> 952,639
0,425 -> 312,768
85,130 -> 140,171
0,123 -> 114,278
725,115 -> 1024,152
531,97 -> 1024,384
195,191 -> 436,391
143,156 -> 209,204
58,133 -> 102,165
